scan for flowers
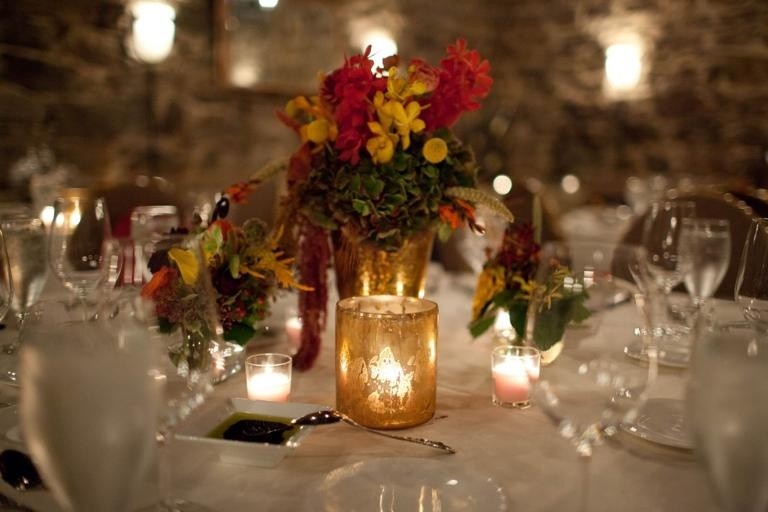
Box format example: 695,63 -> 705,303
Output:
140,35 -> 598,378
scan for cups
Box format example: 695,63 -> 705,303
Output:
18,328 -> 161,512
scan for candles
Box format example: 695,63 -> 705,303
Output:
493,345 -> 539,407
244,352 -> 292,402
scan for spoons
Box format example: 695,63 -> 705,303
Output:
290,408 -> 456,454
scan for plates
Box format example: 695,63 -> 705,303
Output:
171,397 -> 332,467
621,397 -> 698,451
624,327 -> 693,369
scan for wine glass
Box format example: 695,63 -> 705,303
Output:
627,196 -> 766,362
522,274 -> 658,511
0,195 -> 125,383
